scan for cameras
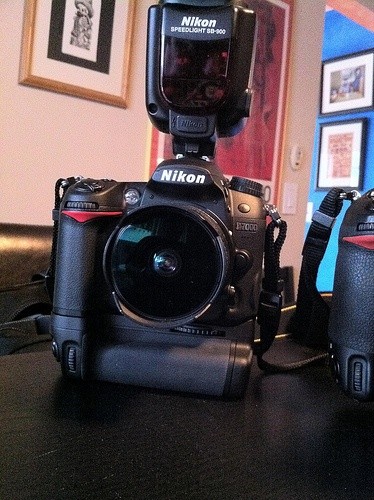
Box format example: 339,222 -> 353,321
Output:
49,0 -> 267,402
328,190 -> 374,402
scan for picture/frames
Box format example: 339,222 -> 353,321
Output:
18,0 -> 138,110
150,0 -> 295,212
318,48 -> 374,117
315,118 -> 368,191
